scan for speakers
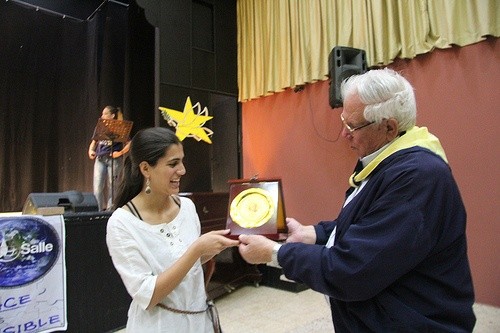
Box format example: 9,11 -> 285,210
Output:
329,46 -> 368,108
22,190 -> 98,216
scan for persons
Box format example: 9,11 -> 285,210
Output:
88,105 -> 133,212
239,68 -> 476,333
105,127 -> 240,333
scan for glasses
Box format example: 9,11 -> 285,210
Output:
338,116 -> 386,136
